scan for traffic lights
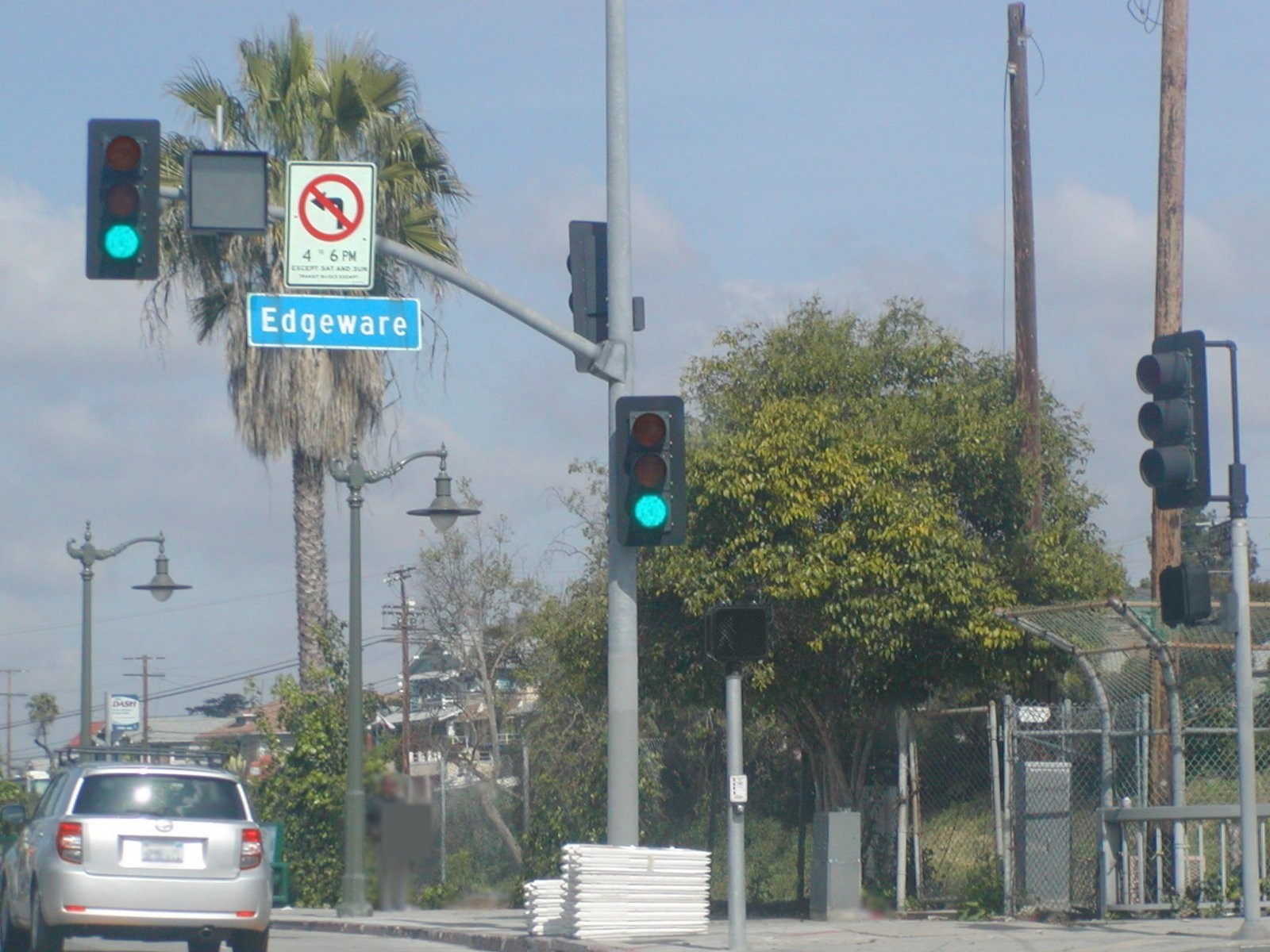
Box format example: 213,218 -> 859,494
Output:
1159,566 -> 1211,629
616,396 -> 688,549
704,605 -> 769,666
1134,329 -> 1211,510
87,117 -> 159,281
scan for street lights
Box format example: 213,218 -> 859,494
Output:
328,431 -> 481,917
67,516 -> 194,766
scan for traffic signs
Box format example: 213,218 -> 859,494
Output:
285,160 -> 378,290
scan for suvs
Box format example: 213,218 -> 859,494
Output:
0,749 -> 271,952
